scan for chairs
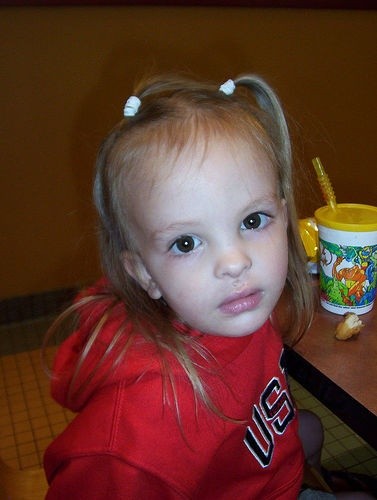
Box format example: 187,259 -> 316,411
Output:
0,458 -> 333,500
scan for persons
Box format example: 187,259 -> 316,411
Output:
43,75 -> 377,500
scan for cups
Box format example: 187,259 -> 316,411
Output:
314,203 -> 377,316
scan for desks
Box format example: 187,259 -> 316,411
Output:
273,274 -> 377,450
